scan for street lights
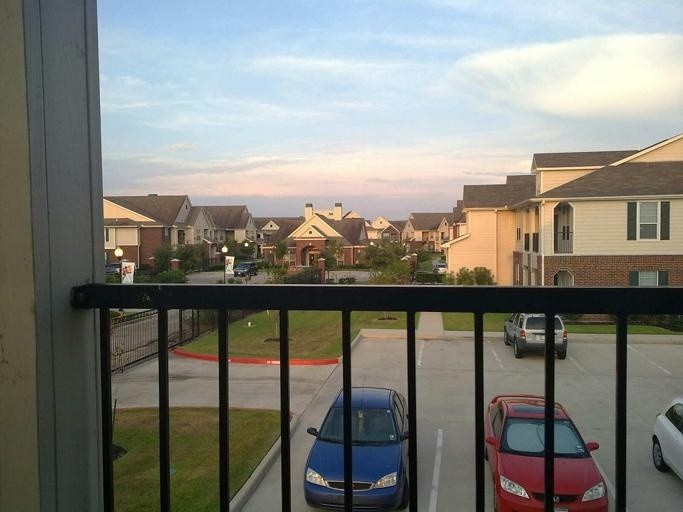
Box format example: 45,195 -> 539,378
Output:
221,244 -> 228,284
114,247 -> 123,312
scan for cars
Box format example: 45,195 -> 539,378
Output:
484,393 -> 608,512
651,395 -> 683,480
435,263 -> 447,273
303,387 -> 409,511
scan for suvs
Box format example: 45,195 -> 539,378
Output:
233,262 -> 257,278
503,311 -> 568,359
